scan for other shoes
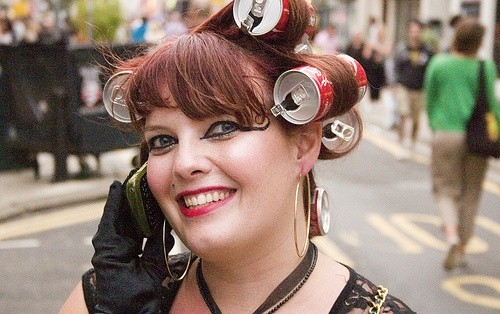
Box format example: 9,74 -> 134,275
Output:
443,239 -> 461,269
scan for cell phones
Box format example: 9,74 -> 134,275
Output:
126,162 -> 164,237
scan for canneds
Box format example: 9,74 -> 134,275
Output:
270,54 -> 367,125
306,188 -> 330,237
233,0 -> 317,42
316,107 -> 363,159
103,69 -> 146,124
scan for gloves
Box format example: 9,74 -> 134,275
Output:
91,139 -> 175,314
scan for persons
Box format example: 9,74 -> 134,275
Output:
60,0 -> 414,314
423,21 -> 499,272
1,0 -> 467,103
395,18 -> 435,145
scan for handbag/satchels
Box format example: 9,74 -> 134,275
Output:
466,60 -> 499,159
